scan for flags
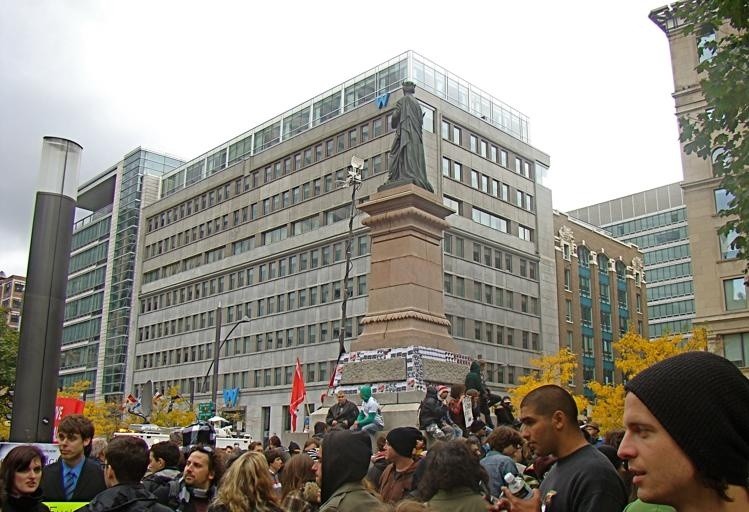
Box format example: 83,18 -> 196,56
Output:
289,358 -> 306,434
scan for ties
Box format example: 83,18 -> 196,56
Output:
65,473 -> 75,499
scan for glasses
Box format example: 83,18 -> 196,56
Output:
203,446 -> 213,453
99,463 -> 108,471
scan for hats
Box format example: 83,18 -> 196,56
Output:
434,385 -> 449,395
386,427 -> 422,455
471,421 -> 485,433
466,389 -> 479,397
586,422 -> 600,433
624,352 -> 748,478
289,442 -> 300,452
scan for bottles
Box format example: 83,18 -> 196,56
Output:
503,472 -> 547,511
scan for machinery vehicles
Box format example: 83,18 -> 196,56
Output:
113,423 -> 252,456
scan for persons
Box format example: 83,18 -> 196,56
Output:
388,80 -> 426,182
41,414 -> 107,512
208,436 -> 323,511
417,359 -> 628,511
327,391 -> 359,430
617,350 -> 748,512
352,387 -> 383,433
0,445 -> 49,512
145,440 -> 219,512
86,434 -> 173,512
302,421 -> 417,511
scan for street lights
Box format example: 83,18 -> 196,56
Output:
11,135 -> 83,446
199,315 -> 251,416
338,156 -> 366,363
